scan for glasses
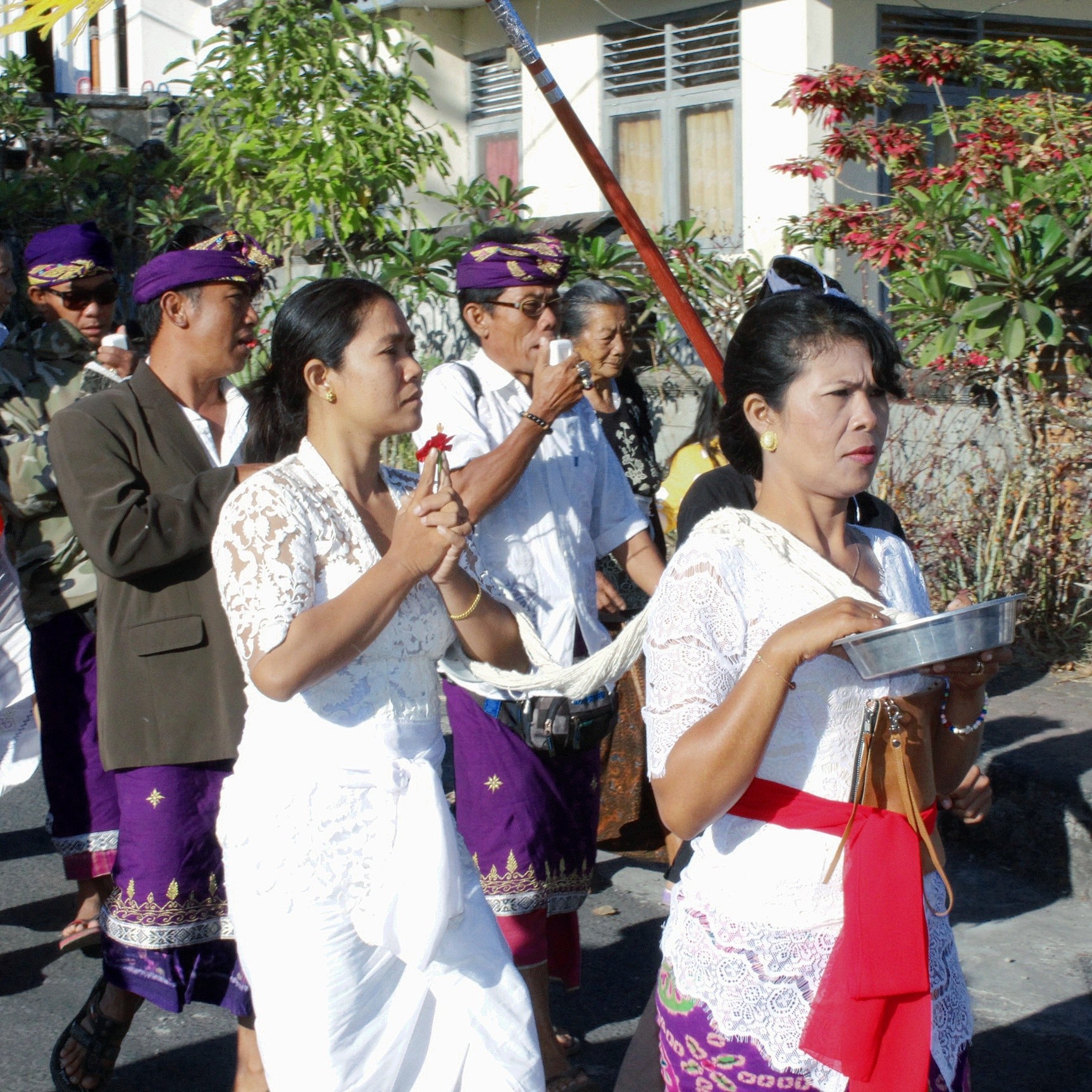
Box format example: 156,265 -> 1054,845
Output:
40,279 -> 120,313
477,298 -> 568,318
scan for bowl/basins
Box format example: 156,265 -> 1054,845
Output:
828,592 -> 1027,680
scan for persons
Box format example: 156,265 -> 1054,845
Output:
207,279 -> 547,1092
1,224 -> 995,1092
643,290 -> 994,1092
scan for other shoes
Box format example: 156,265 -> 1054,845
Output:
552,1025 -> 582,1055
546,1064 -> 591,1092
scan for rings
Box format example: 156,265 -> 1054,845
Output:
971,661 -> 985,677
110,368 -> 117,375
871,613 -> 879,619
575,360 -> 590,378
581,377 -> 595,391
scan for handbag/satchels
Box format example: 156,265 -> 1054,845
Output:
467,684 -> 617,761
820,689 -> 954,917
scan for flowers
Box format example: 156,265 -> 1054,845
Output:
416,424 -> 458,494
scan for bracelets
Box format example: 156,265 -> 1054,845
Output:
758,652 -> 797,692
518,411 -> 549,431
937,678 -> 988,736
450,584 -> 482,619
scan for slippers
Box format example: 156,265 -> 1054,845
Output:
58,913 -> 106,951
49,973 -> 146,1092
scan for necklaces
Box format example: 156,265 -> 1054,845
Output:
847,532 -> 861,582
